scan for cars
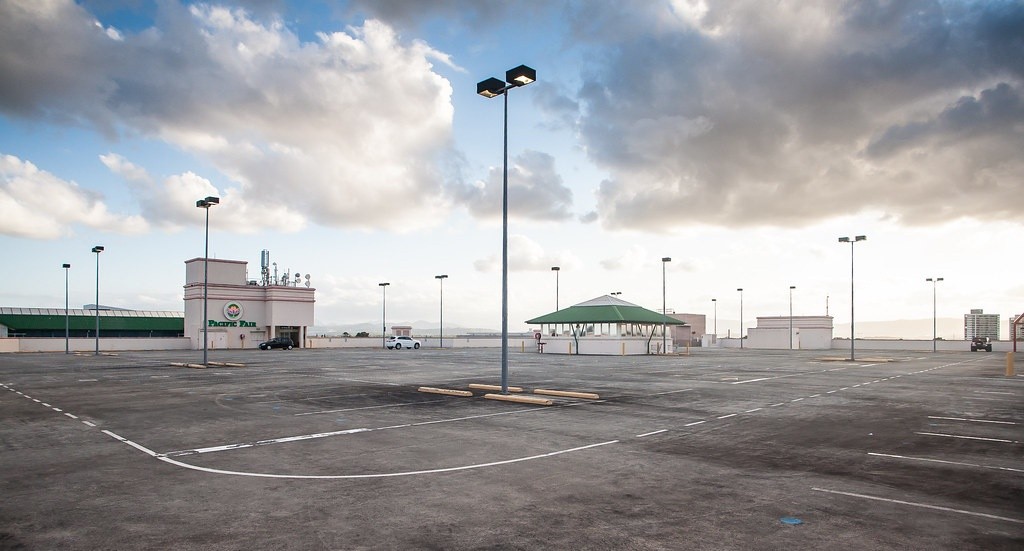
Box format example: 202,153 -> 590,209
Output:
258,337 -> 295,351
92,245 -> 104,358
386,336 -> 422,350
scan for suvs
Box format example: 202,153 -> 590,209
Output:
971,336 -> 992,352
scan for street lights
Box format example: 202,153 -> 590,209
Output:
435,275 -> 448,348
550,266 -> 560,312
837,235 -> 867,361
789,286 -> 796,350
196,195 -> 220,369
661,257 -> 671,353
62,263 -> 71,353
737,288 -> 744,349
712,298 -> 717,343
926,277 -> 944,352
477,64 -> 537,396
378,282 -> 390,349
